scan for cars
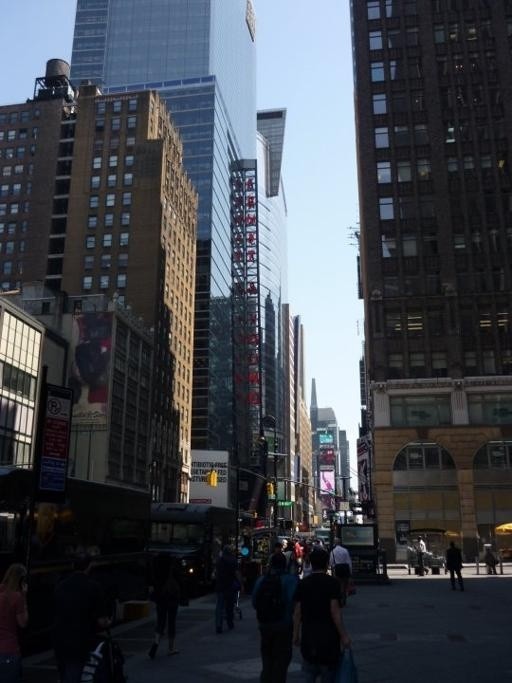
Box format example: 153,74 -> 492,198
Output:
308,510 -> 354,555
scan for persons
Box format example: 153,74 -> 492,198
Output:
52,552 -> 113,681
2,564 -> 28,681
486,548 -> 497,575
149,554 -> 188,658
407,535 -> 428,575
212,528 -> 351,682
444,541 -> 464,591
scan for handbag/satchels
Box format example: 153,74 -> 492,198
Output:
334,563 -> 350,577
334,646 -> 360,682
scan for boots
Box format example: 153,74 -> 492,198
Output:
147,632 -> 159,658
166,638 -> 180,655
450,575 -> 464,592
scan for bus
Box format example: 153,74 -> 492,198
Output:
149,502 -> 254,594
0,465 -> 153,632
